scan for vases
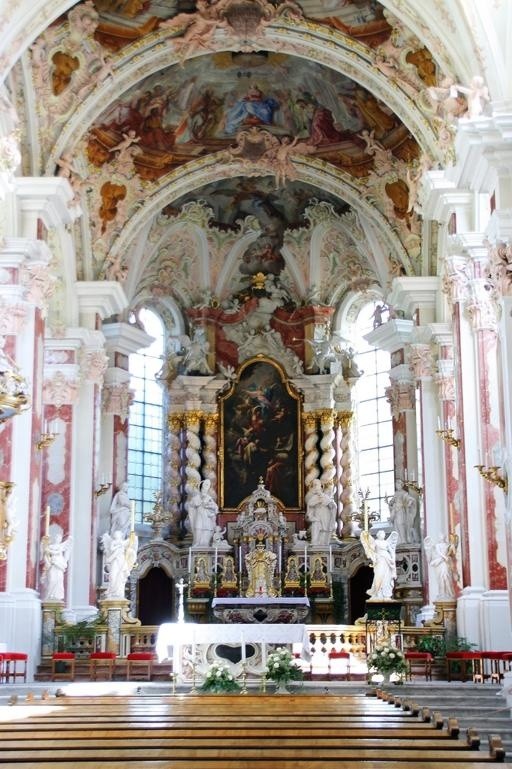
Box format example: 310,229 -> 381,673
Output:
274,678 -> 292,696
378,672 -> 401,684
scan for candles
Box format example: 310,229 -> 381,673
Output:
239,546 -> 242,573
37,413 -> 62,450
188,546 -> 192,574
432,411 -> 509,495
412,469 -> 415,481
130,501 -> 136,533
44,506 -> 49,536
278,545 -> 282,573
449,501 -> 455,535
364,502 -> 369,532
214,546 -> 217,573
305,547 -> 307,571
405,468 -> 407,481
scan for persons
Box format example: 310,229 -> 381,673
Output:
370,530 -> 395,598
309,487 -> 337,544
235,383 -> 288,491
372,305 -> 386,329
197,559 -> 208,582
313,559 -> 323,580
110,482 -> 129,536
223,560 -> 235,581
285,559 -> 299,581
392,480 -> 416,544
244,541 -> 277,598
111,530 -> 138,601
45,535 -> 73,601
190,488 -> 218,547
436,534 -> 456,597
213,525 -> 228,546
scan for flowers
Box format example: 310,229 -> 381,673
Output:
365,635 -> 407,668
195,661 -> 243,693
264,646 -> 300,681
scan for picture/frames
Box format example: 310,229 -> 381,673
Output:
216,352 -> 305,512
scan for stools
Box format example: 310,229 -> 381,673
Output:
443,646 -> 512,687
89,651 -> 115,681
51,652 -> 75,682
125,651 -> 155,683
0,651 -> 10,687
327,652 -> 354,682
404,653 -> 432,684
5,651 -> 30,684
